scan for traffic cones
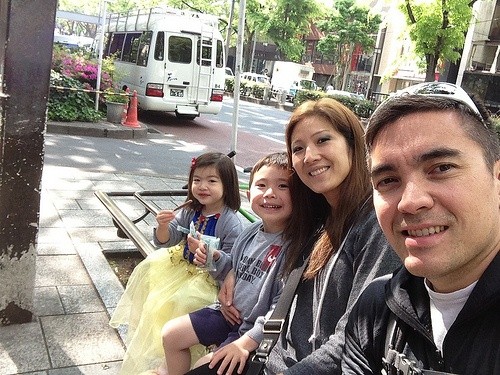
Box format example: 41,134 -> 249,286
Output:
120,87 -> 141,127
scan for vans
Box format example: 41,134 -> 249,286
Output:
239,73 -> 270,93
225,66 -> 236,87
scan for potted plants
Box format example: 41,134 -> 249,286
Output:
103,87 -> 130,124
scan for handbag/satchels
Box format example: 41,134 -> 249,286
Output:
182,351 -> 266,375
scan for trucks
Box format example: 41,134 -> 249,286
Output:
270,61 -> 316,103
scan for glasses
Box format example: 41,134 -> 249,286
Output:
367,81 -> 489,130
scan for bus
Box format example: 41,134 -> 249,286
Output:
101,6 -> 225,120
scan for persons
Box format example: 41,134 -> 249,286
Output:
326,83 -> 334,91
341,81 -> 500,375
261,69 -> 270,77
162,152 -> 303,375
187,97 -> 403,375
109,153 -> 246,375
